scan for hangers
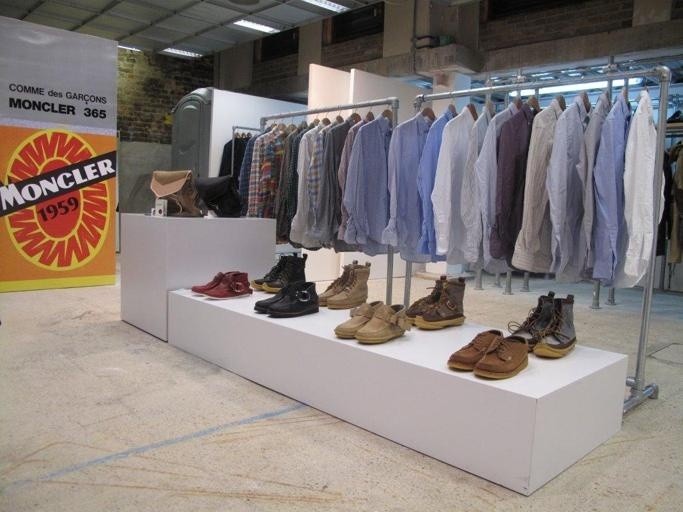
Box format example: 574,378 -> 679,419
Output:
233,97 -> 395,143
395,73 -> 660,143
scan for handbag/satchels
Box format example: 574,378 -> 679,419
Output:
151,169 -> 205,218
193,172 -> 242,217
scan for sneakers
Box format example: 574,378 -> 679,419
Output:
355,304 -> 410,344
203,273 -> 251,299
447,328 -> 501,370
268,283 -> 318,317
475,336 -> 530,380
191,270 -> 241,294
255,284 -> 305,312
334,301 -> 384,338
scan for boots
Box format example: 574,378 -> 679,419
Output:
415,280 -> 466,330
534,298 -> 580,357
251,255 -> 296,290
318,264 -> 360,306
327,265 -> 370,309
512,294 -> 554,352
405,277 -> 454,323
263,256 -> 306,293
